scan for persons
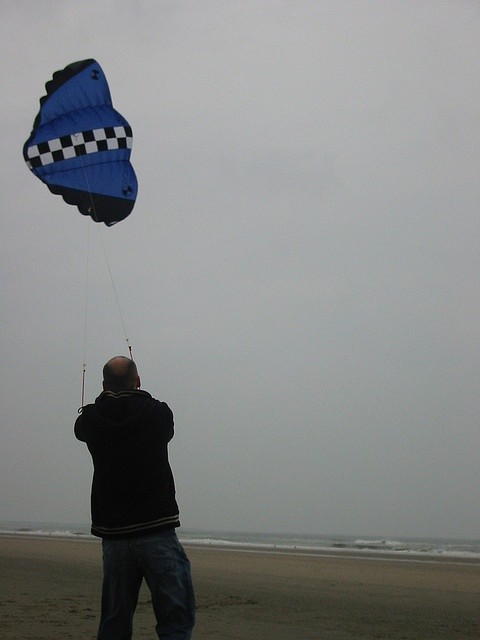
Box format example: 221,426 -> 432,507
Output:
73,357 -> 197,639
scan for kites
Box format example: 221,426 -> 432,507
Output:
22,58 -> 139,227
